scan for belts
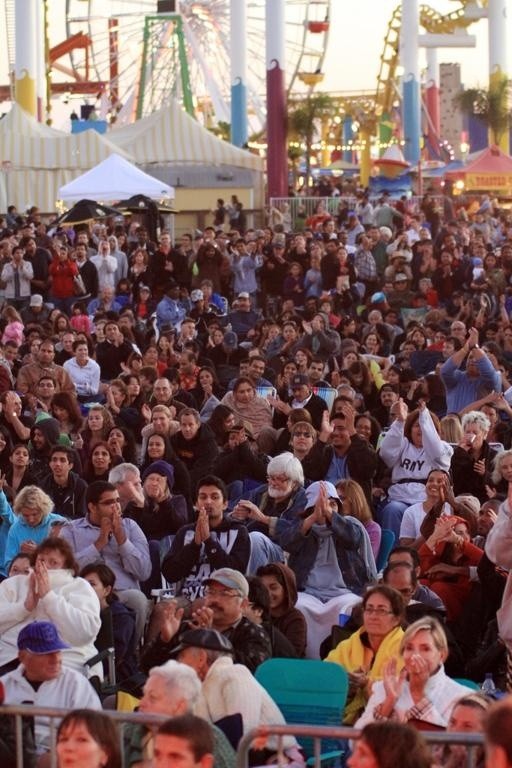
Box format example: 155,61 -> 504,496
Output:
390,477 -> 428,486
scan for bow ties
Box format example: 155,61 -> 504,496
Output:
14,267 -> 21,297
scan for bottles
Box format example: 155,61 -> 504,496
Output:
480,672 -> 496,694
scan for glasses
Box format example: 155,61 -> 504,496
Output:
450,327 -> 464,332
395,280 -> 406,284
292,431 -> 313,438
338,494 -> 347,501
362,608 -> 393,617
257,236 -> 265,240
434,335 -> 445,340
202,586 -> 241,599
283,320 -> 297,329
265,475 -> 289,483
96,498 -> 120,506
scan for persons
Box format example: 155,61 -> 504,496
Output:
1,172 -> 511,768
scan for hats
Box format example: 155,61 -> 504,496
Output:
163,277 -> 180,292
370,291 -> 385,304
17,620 -> 72,656
394,274 -> 407,282
141,460 -> 175,490
304,480 -> 343,512
225,419 -> 256,441
191,289 -> 204,303
202,567 -> 250,599
168,628 -> 234,656
389,252 -> 407,263
292,375 -> 310,390
239,292 -> 250,300
139,285 -> 151,293
273,242 -> 285,249
29,294 -> 43,308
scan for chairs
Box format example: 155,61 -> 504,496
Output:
254,387 -> 277,420
84,610 -> 119,694
376,529 -> 398,571
288,387 -> 338,413
254,656 -> 351,766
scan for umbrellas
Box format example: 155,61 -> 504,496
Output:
55,148 -> 177,206
